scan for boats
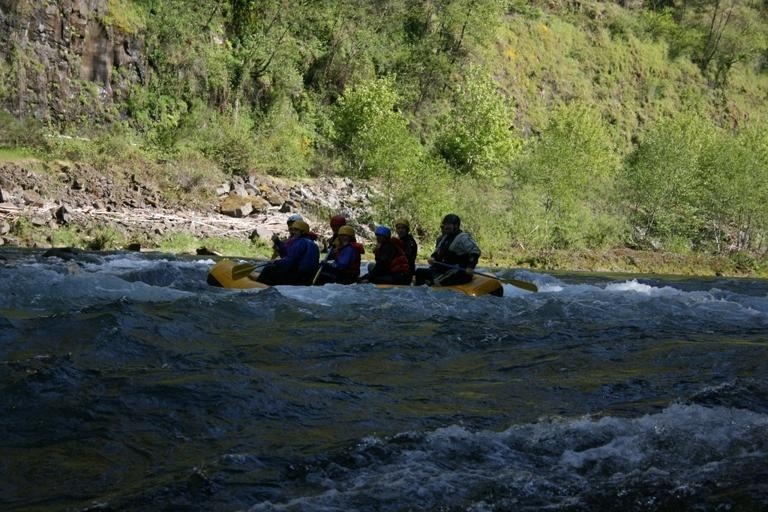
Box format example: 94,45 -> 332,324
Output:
206,259 -> 503,302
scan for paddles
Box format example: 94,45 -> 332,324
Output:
272,239 -> 289,260
232,263 -> 272,281
433,262 -> 537,292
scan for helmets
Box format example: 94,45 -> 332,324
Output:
330,215 -> 345,226
440,214 -> 460,227
287,214 -> 309,232
375,226 -> 391,238
338,226 -> 355,238
396,218 -> 409,227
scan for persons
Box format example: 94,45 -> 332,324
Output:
411,213 -> 481,286
326,215 -> 347,260
270,214 -> 307,257
394,218 -> 418,269
367,225 -> 413,286
254,219 -> 320,285
314,225 -> 362,284
435,216 -> 449,248
